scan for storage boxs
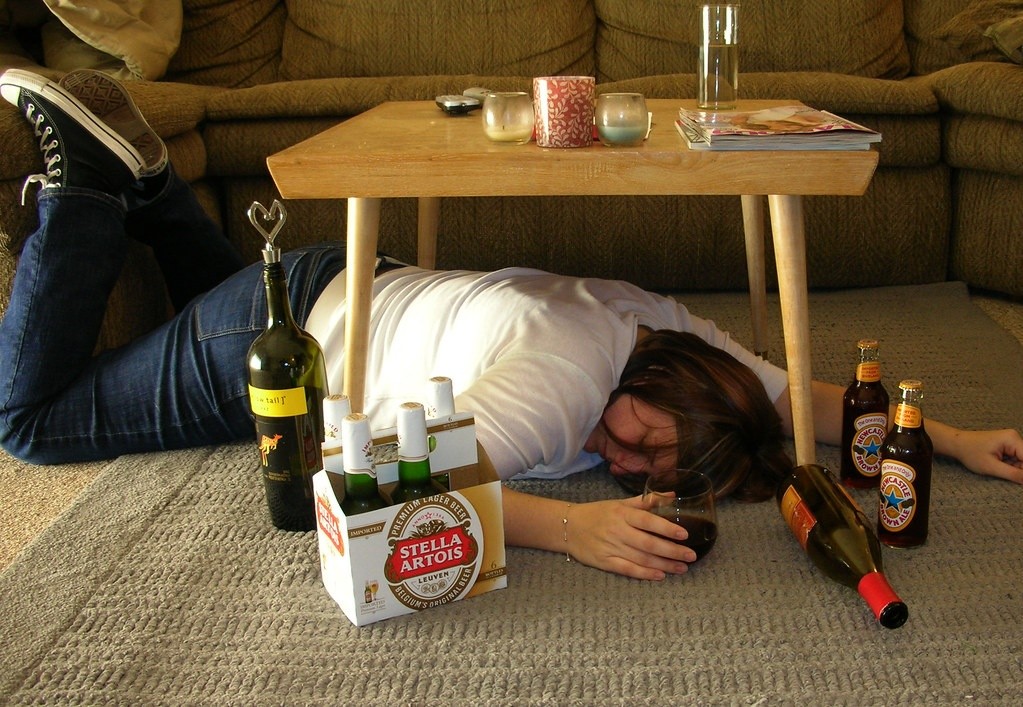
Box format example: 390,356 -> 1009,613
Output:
312,409 -> 507,626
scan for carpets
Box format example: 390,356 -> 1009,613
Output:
1,282 -> 1023,707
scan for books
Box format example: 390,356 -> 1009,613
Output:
674,102 -> 883,154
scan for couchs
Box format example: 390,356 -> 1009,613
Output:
0,3 -> 1022,394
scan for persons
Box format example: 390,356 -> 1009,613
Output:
0,66 -> 1023,581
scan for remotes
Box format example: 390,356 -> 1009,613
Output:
436,94 -> 481,115
464,87 -> 493,105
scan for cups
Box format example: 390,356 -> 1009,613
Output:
482,91 -> 534,146
594,92 -> 649,148
697,3 -> 741,111
641,468 -> 719,563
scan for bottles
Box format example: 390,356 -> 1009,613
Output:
244,262 -> 332,532
388,402 -> 451,505
877,379 -> 934,550
839,338 -> 890,491
322,393 -> 390,516
775,463 -> 909,630
425,376 -> 456,421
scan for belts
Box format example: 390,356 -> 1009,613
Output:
304,256 -> 409,340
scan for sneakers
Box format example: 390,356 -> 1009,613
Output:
1,68 -> 147,205
59,69 -> 170,182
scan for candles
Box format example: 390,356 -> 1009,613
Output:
484,91 -> 534,145
532,76 -> 595,148
594,93 -> 648,142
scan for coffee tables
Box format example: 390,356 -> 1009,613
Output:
265,99 -> 879,479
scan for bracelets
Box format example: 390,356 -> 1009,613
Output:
563,503 -> 572,562
